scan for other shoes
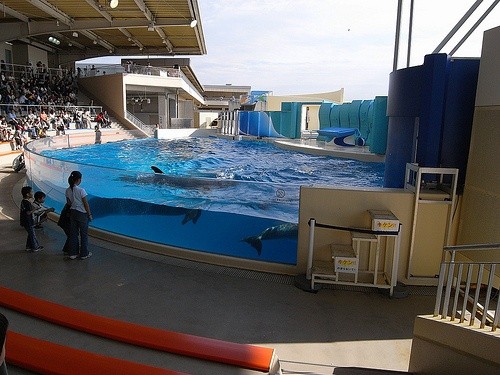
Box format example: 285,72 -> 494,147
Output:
80,252 -> 92,259
69,254 -> 78,259
33,246 -> 44,251
26,248 -> 33,250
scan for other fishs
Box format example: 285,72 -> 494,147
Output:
86,166 -> 243,225
242,223 -> 298,255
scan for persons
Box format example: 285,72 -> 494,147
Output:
33,191 -> 55,229
204,94 -> 232,100
0,60 -> 112,143
20,186 -> 44,251
14,158 -> 23,172
10,131 -> 26,150
56,171 -> 93,259
125,60 -> 152,75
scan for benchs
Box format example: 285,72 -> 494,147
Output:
17,122 -> 116,137
0,285 -> 282,375
